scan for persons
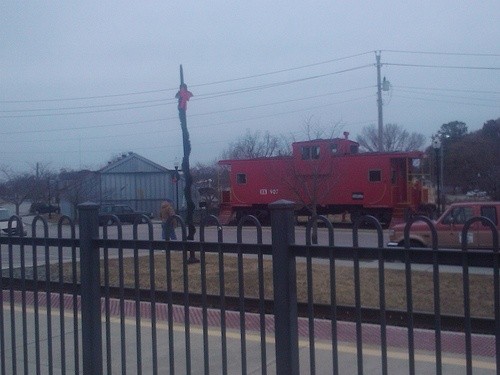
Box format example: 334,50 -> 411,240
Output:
159,201 -> 178,253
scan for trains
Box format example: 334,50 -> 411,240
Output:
214,132 -> 425,227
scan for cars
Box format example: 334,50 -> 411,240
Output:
98,204 -> 155,225
29,203 -> 59,215
0,208 -> 28,237
386,201 -> 500,263
